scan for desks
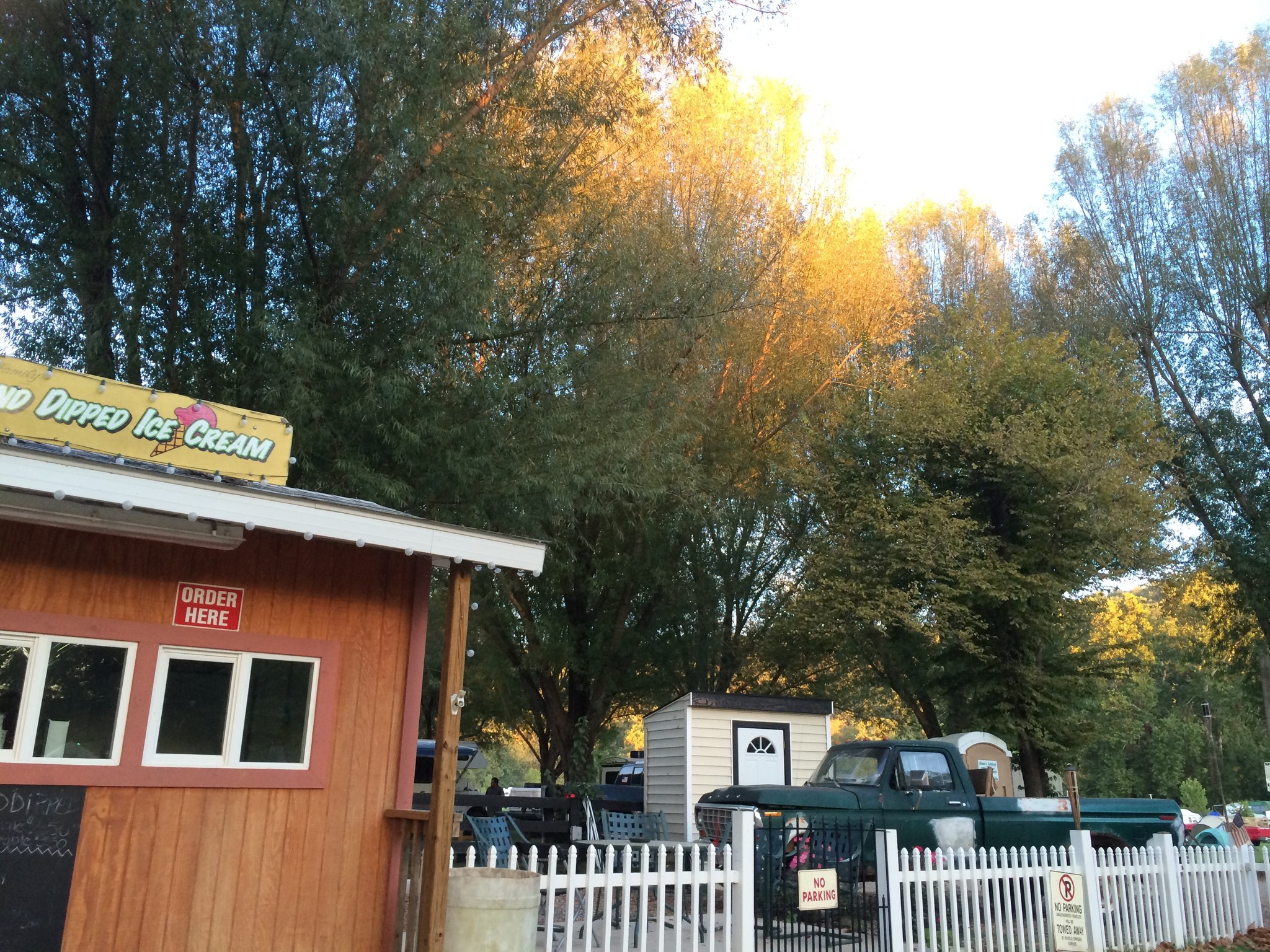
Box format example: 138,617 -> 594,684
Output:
573,837 -> 714,948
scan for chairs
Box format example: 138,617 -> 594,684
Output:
711,820 -> 792,939
465,813 -> 591,940
601,808 -> 692,928
772,816 -> 872,948
700,821 -> 792,937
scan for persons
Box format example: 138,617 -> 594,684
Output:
485,778 -> 505,796
457,806 -> 488,863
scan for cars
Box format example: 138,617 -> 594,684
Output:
1179,800 -> 1270,847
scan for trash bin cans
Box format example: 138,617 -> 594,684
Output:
444,867 -> 541,952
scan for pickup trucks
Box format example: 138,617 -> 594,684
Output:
503,750 -> 644,862
693,739 -> 1186,909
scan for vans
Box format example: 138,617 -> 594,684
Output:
412,739 -> 492,838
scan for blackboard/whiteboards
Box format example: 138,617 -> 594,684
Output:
0,783 -> 86,952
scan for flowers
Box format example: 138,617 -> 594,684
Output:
913,845 -> 946,865
789,835 -> 844,872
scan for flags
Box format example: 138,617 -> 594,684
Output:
1230,813 -> 1250,848
